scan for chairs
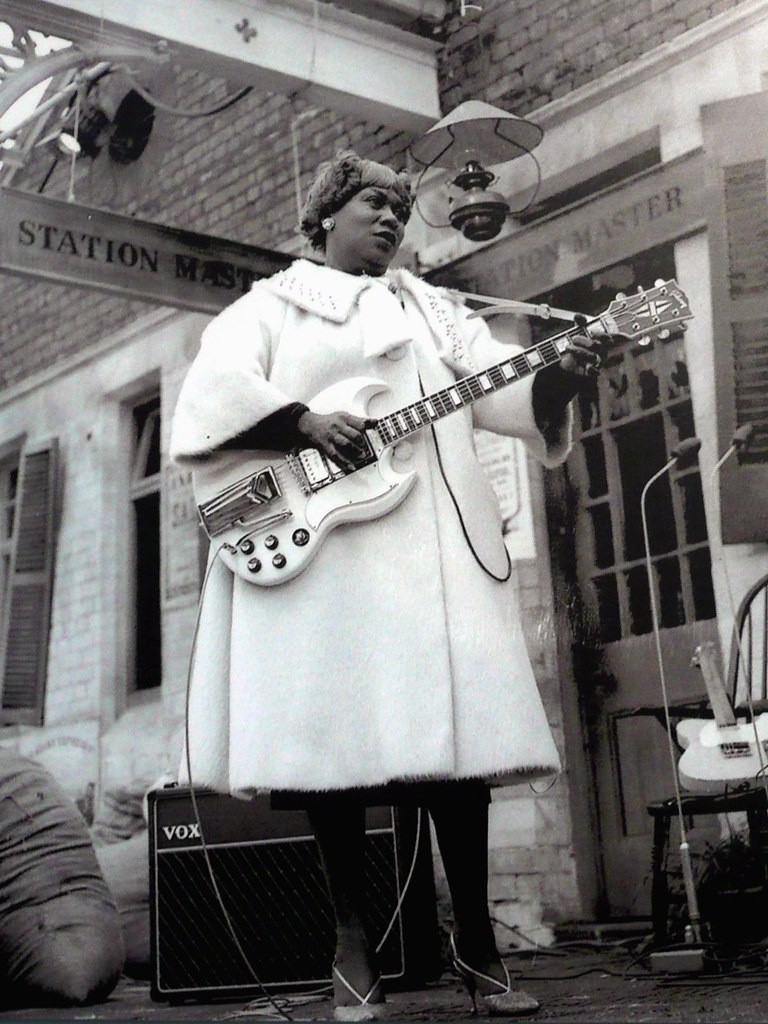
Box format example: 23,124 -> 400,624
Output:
629,573 -> 768,978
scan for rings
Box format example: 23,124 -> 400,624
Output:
593,354 -> 601,368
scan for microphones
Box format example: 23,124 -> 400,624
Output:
672,437 -> 701,464
730,422 -> 756,451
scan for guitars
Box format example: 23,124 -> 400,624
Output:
674,641 -> 767,795
187,280 -> 696,585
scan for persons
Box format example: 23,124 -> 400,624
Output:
166,150 -> 613,1022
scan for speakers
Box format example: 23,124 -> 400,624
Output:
147,780 -> 442,1006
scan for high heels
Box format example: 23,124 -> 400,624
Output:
448,929 -> 541,1017
331,958 -> 388,1023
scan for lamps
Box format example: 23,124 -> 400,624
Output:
408,0 -> 544,243
60,88 -> 155,163
